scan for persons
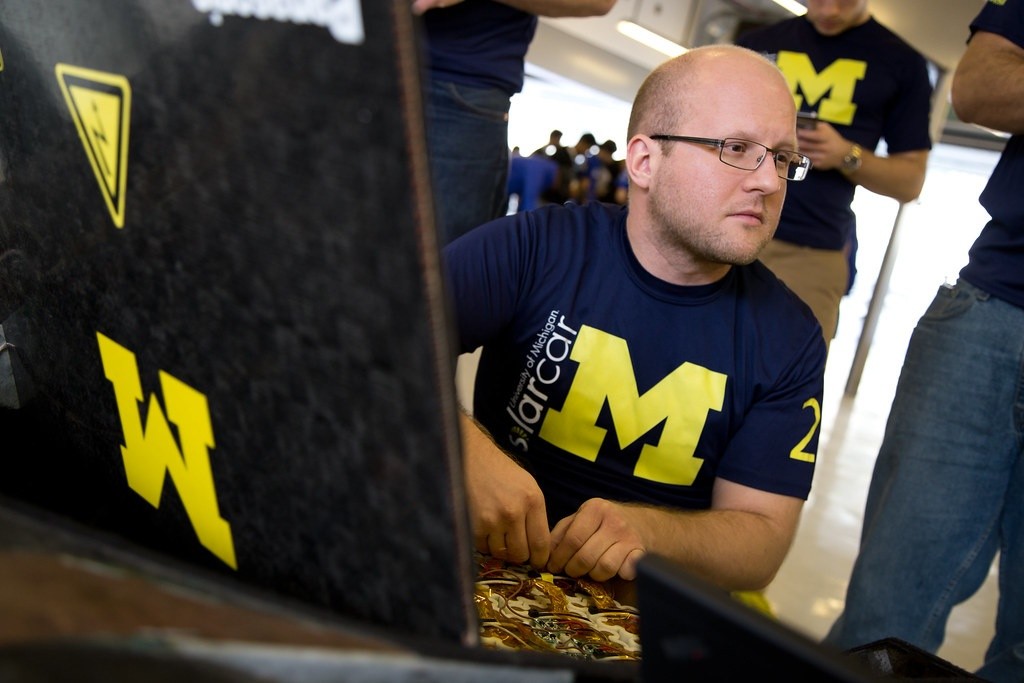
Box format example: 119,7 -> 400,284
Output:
740,0 -> 933,351
409,0 -> 614,246
505,130 -> 629,217
438,43 -> 827,595
819,0 -> 1024,683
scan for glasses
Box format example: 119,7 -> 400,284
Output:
647,135 -> 813,182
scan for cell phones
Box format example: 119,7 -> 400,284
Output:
795,111 -> 818,130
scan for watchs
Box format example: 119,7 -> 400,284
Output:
840,144 -> 862,174
